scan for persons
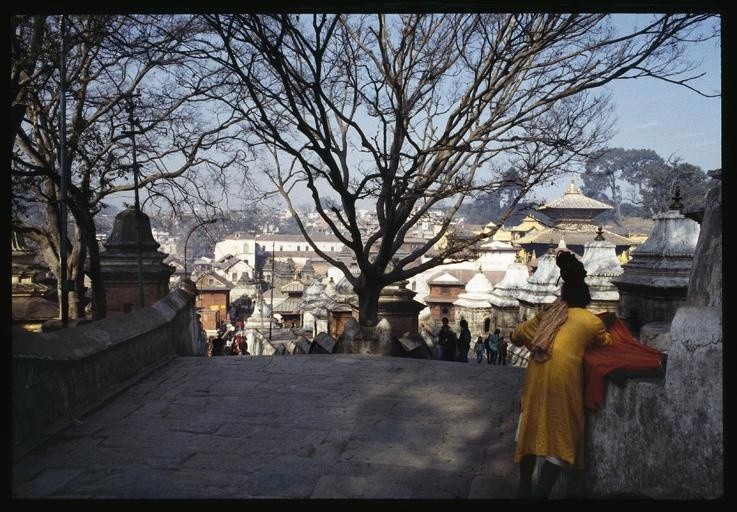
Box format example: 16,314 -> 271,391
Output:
510,251 -> 613,500
439,317 -> 508,366
212,318 -> 248,356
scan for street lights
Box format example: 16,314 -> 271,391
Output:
185,216 -> 218,279
